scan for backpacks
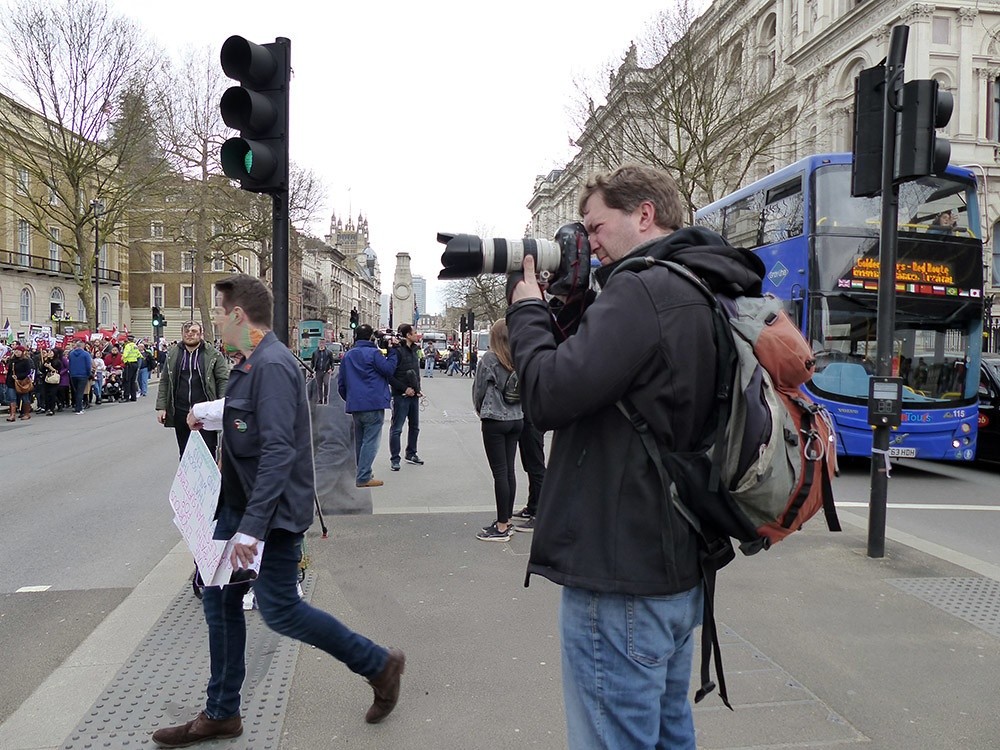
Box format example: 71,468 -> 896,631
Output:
491,365 -> 521,405
590,256 -> 842,556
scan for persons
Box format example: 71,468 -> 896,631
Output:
388,324 -> 424,471
155,320 -> 230,464
52,313 -> 61,321
465,347 -> 478,376
913,357 -> 929,389
937,358 -> 964,393
214,339 -> 234,364
512,423 -> 546,532
337,324 -> 400,487
308,339 -> 334,405
153,275 -> 406,747
900,355 -> 912,386
423,341 -> 436,378
64,312 -> 72,320
442,347 -> 465,377
0,335 -> 178,422
472,318 -> 524,542
508,165 -> 767,750
926,212 -> 958,235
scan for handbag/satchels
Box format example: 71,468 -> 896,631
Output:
45,370 -> 61,384
14,375 -> 34,395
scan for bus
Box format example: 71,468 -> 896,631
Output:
298,320 -> 325,361
590,153 -> 989,463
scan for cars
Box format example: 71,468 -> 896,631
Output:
910,352 -> 1000,462
326,342 -> 345,364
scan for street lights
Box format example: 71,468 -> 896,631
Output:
187,246 -> 198,320
90,199 -> 103,334
297,292 -> 304,319
55,306 -> 63,335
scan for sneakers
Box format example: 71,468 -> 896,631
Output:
511,507 -> 530,520
476,526 -> 511,542
515,516 -> 536,533
482,521 -> 515,536
152,710 -> 243,748
365,645 -> 406,722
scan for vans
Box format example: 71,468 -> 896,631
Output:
418,333 -> 450,369
458,331 -> 489,365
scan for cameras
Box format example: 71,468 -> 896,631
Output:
437,222 -> 590,297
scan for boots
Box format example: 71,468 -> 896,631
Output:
6,402 -> 16,421
21,402 -> 31,420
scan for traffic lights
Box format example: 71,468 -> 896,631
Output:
349,310 -> 359,329
460,317 -> 468,332
892,78 -> 954,185
159,314 -> 168,327
151,306 -> 161,327
220,34 -> 289,192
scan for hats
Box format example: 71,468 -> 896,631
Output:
127,335 -> 135,339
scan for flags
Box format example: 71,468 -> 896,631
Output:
111,323 -> 128,337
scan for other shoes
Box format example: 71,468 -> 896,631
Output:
72,408 -> 75,411
390,460 -> 400,470
95,391 -> 149,404
355,477 -> 383,487
405,455 -> 424,465
46,411 -> 54,416
85,404 -> 90,409
76,411 -> 85,415
58,405 -> 62,412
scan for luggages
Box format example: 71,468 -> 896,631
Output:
36,409 -> 46,414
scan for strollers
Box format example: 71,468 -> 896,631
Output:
101,366 -> 124,402
